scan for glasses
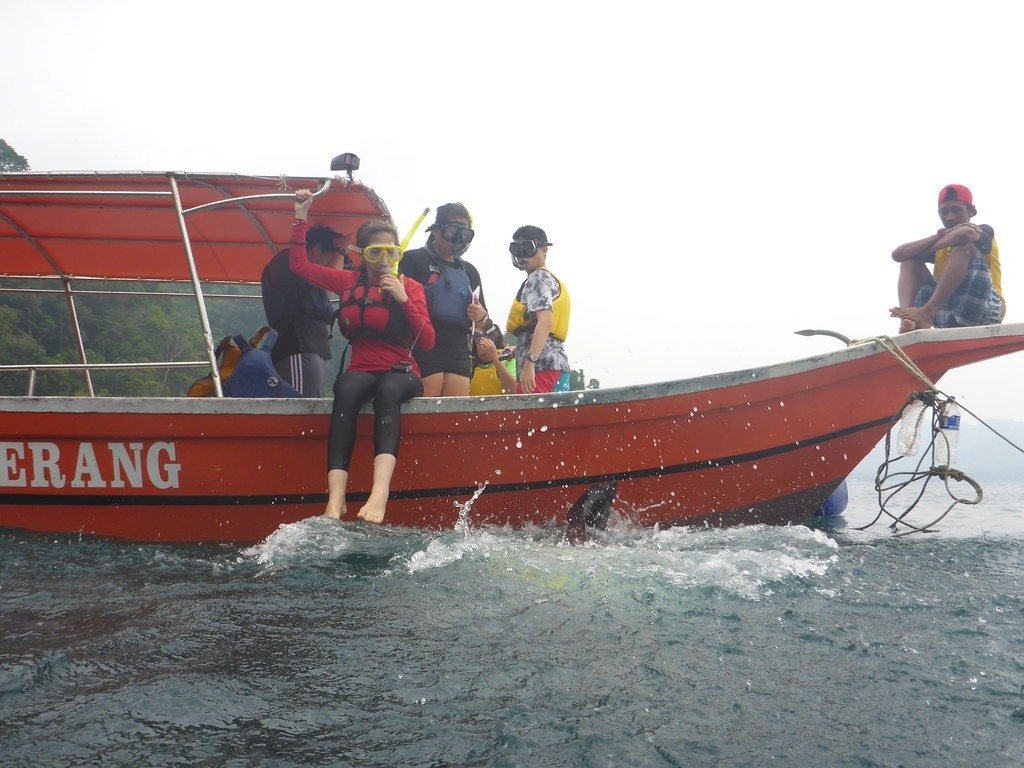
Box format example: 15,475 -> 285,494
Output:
361,242 -> 404,266
508,241 -> 552,259
435,220 -> 474,244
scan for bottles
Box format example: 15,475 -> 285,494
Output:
933,395 -> 962,465
897,397 -> 927,457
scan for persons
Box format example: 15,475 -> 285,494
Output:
892,185 -> 1005,332
259,220 -> 353,397
470,319 -> 517,396
290,190 -> 435,526
505,225 -> 571,394
399,202 -> 489,396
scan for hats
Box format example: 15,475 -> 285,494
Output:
937,184 -> 978,218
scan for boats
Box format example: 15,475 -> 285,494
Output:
2,164 -> 1023,545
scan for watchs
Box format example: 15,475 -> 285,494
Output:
524,353 -> 538,362
482,312 -> 488,323
332,247 -> 348,258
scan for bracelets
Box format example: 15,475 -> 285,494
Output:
937,228 -> 945,237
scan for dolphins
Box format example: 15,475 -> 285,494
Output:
567,479 -> 617,550
